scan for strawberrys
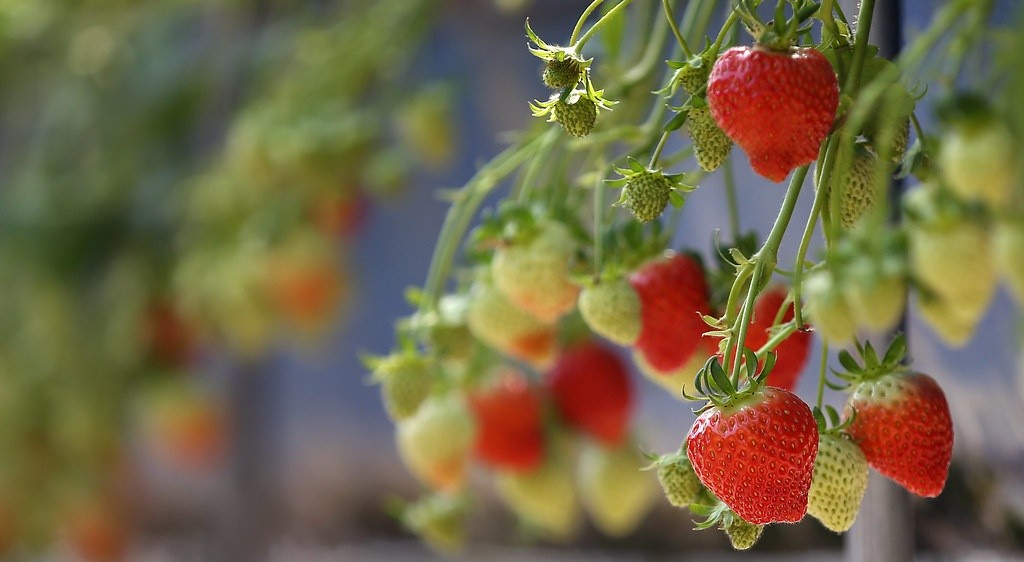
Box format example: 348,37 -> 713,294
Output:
0,24 -> 1024,561
